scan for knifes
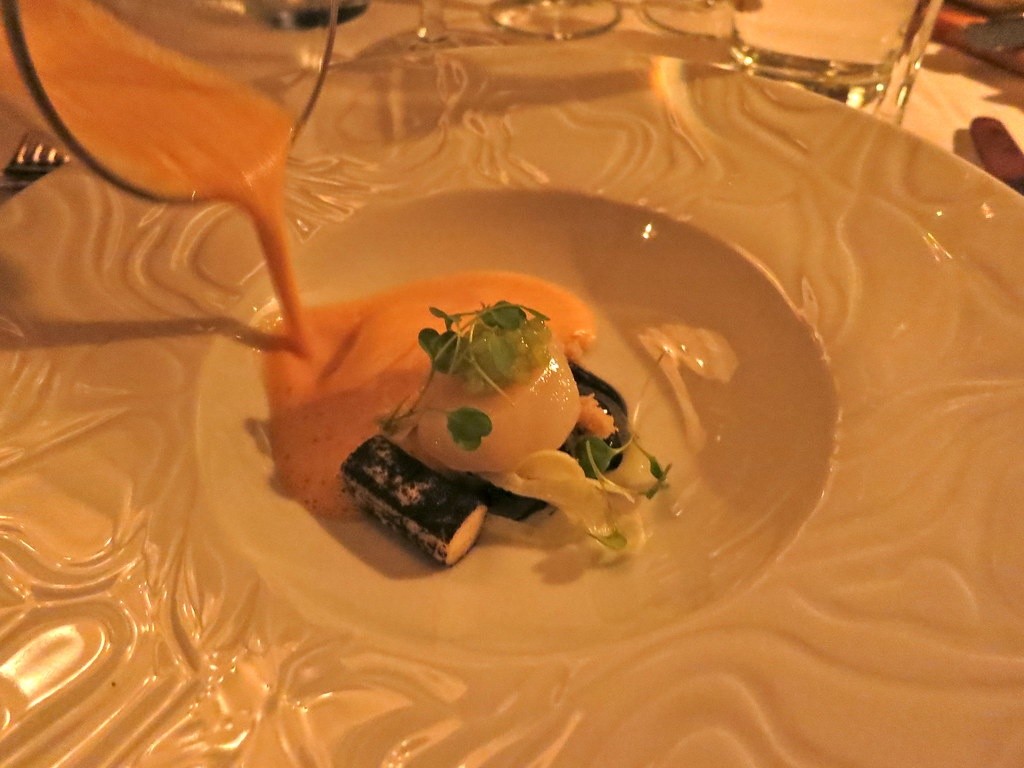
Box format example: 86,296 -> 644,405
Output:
970,118 -> 1023,196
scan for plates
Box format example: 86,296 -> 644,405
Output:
0,47 -> 1024,768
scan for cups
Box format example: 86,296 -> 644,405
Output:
1,1 -> 338,204
724,0 -> 946,126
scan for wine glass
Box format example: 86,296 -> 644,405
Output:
355,0 -> 508,71
